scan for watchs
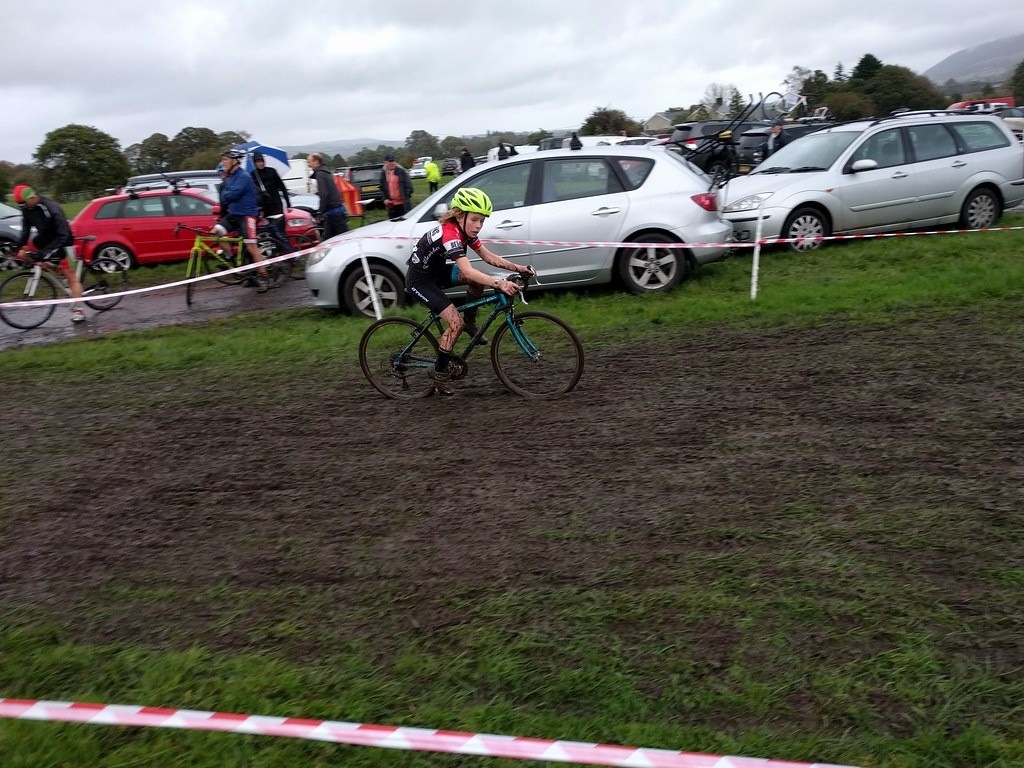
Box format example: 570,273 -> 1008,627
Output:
494,278 -> 502,288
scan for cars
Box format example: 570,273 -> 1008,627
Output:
990,107 -> 1024,134
0,202 -> 39,271
409,163 -> 427,179
66,190 -> 321,275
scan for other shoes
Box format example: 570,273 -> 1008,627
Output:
71,306 -> 86,321
216,259 -> 236,269
256,278 -> 274,293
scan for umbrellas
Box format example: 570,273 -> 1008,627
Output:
219,140 -> 291,181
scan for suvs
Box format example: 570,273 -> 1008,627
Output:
441,159 -> 457,176
666,119 -> 776,181
303,145 -> 732,318
334,163 -> 387,209
732,121 -> 835,177
118,169 -> 320,220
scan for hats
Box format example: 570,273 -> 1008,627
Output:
253,152 -> 264,163
13,185 -> 35,202
385,154 -> 395,162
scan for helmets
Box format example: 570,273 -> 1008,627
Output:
771,119 -> 782,126
218,163 -> 225,171
220,149 -> 244,159
450,188 -> 492,217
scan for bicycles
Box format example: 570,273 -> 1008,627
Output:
359,265 -> 584,400
174,199 -> 375,307
0,240 -> 128,330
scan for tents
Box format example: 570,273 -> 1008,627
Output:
332,176 -> 364,215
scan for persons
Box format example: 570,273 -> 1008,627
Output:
765,117 -> 794,159
423,132 -> 583,195
10,184 -> 87,323
404,187 -> 535,397
379,153 -> 414,219
210,150 -> 293,293
306,152 -> 350,239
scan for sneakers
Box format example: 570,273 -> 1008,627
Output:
464,319 -> 488,346
427,363 -> 456,397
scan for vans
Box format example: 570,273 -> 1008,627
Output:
716,110 -> 1024,252
487,135 -> 661,180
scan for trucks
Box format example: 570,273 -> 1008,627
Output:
946,97 -> 1015,110
